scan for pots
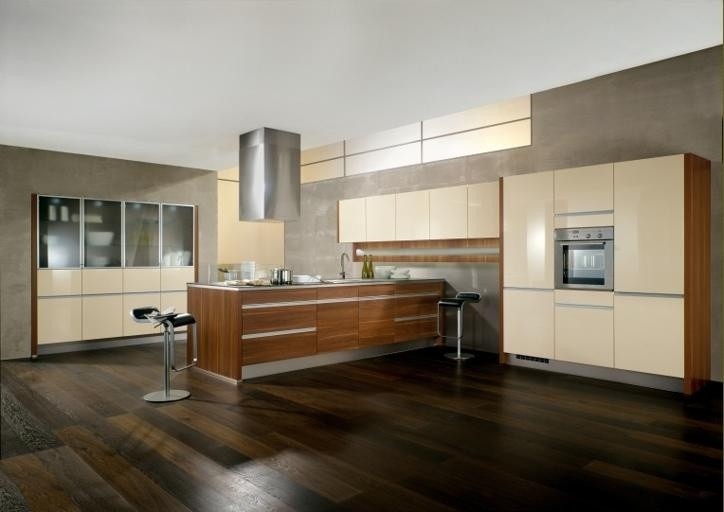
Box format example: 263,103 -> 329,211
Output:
271,267 -> 292,284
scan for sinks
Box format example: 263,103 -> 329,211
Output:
328,278 -> 385,284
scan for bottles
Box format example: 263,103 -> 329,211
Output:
48,204 -> 70,222
361,254 -> 374,280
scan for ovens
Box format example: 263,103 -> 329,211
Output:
552,226 -> 616,292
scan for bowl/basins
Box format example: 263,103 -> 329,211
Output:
292,275 -> 311,282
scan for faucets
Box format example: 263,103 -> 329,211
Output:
340,252 -> 351,278
